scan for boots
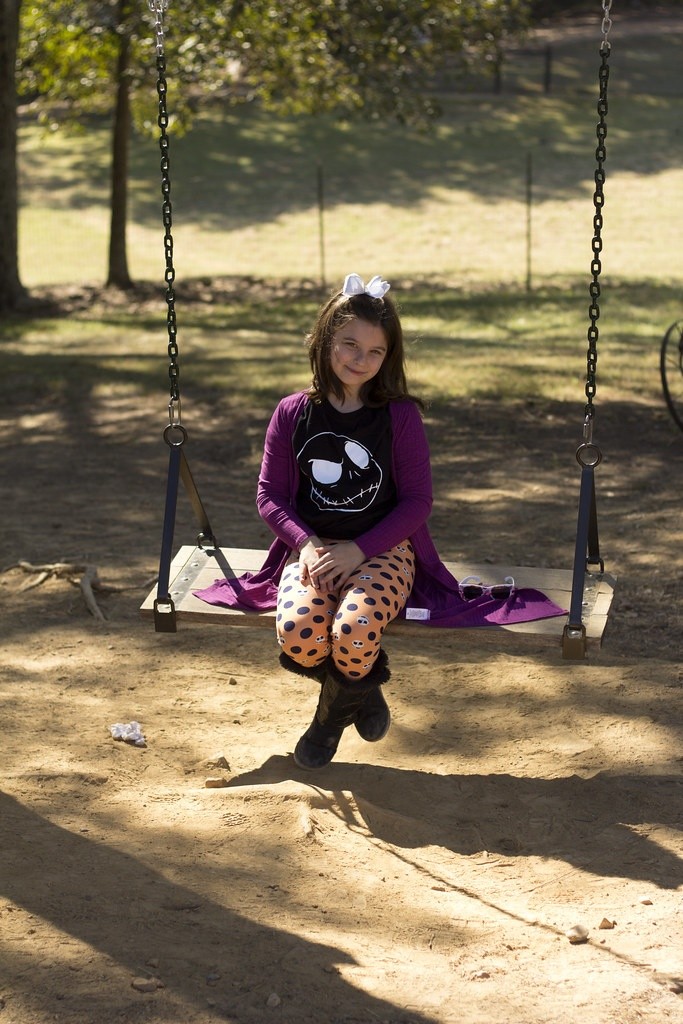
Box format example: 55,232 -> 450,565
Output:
278,649 -> 391,771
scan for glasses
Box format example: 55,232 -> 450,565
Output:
459,576 -> 514,603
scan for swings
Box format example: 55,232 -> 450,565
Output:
139,1 -> 619,659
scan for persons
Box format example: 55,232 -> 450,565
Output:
192,274 -> 569,769
